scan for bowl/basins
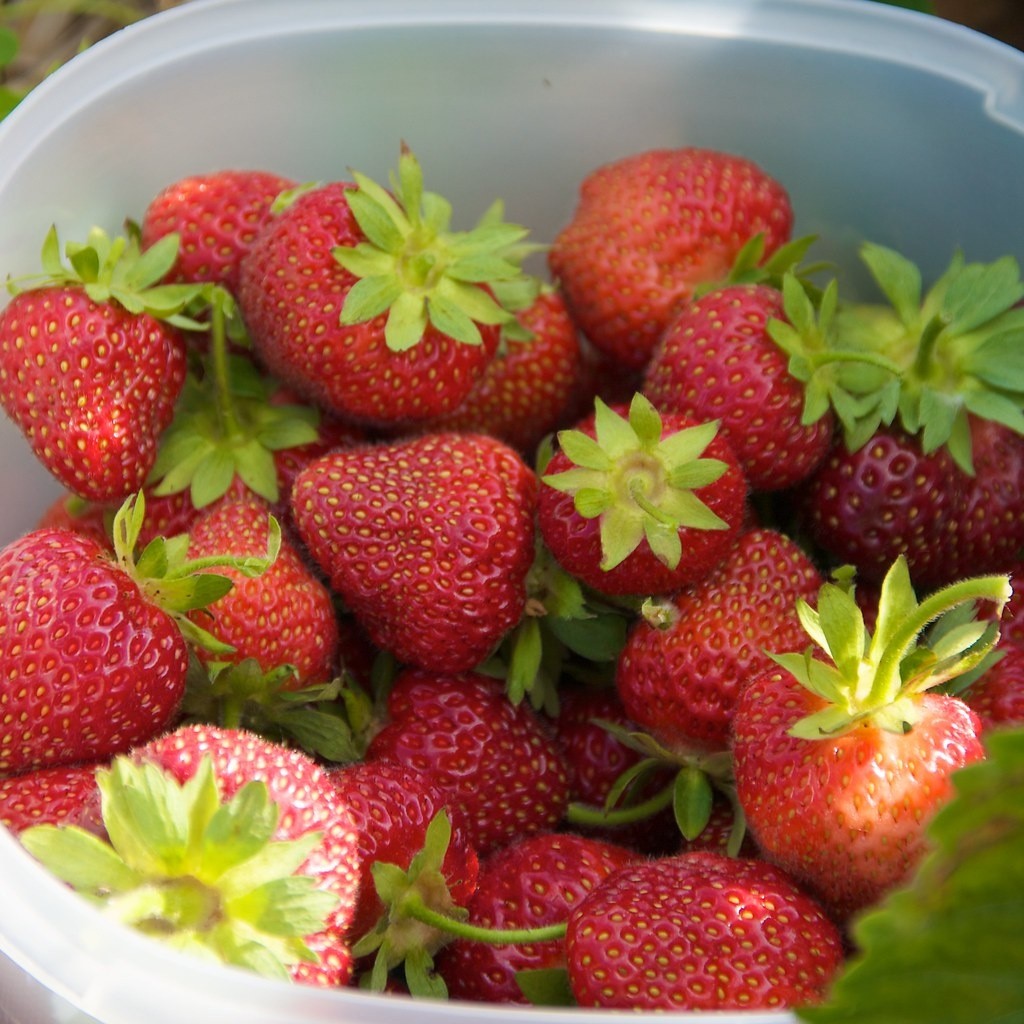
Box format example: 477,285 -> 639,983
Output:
0,0 -> 1024,1024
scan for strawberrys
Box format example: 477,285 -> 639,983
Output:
1,147 -> 1024,1024
